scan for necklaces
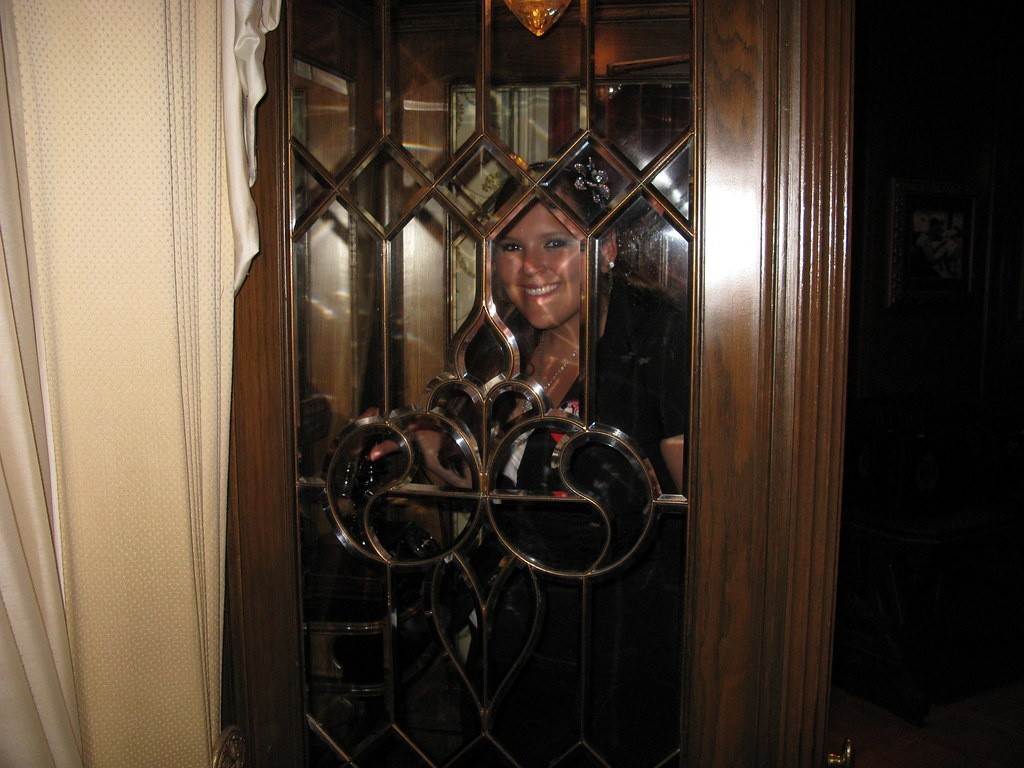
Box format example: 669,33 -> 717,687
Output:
523,329 -> 580,415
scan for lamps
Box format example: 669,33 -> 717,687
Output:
502,0 -> 574,36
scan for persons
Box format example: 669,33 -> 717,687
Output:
366,162 -> 690,768
